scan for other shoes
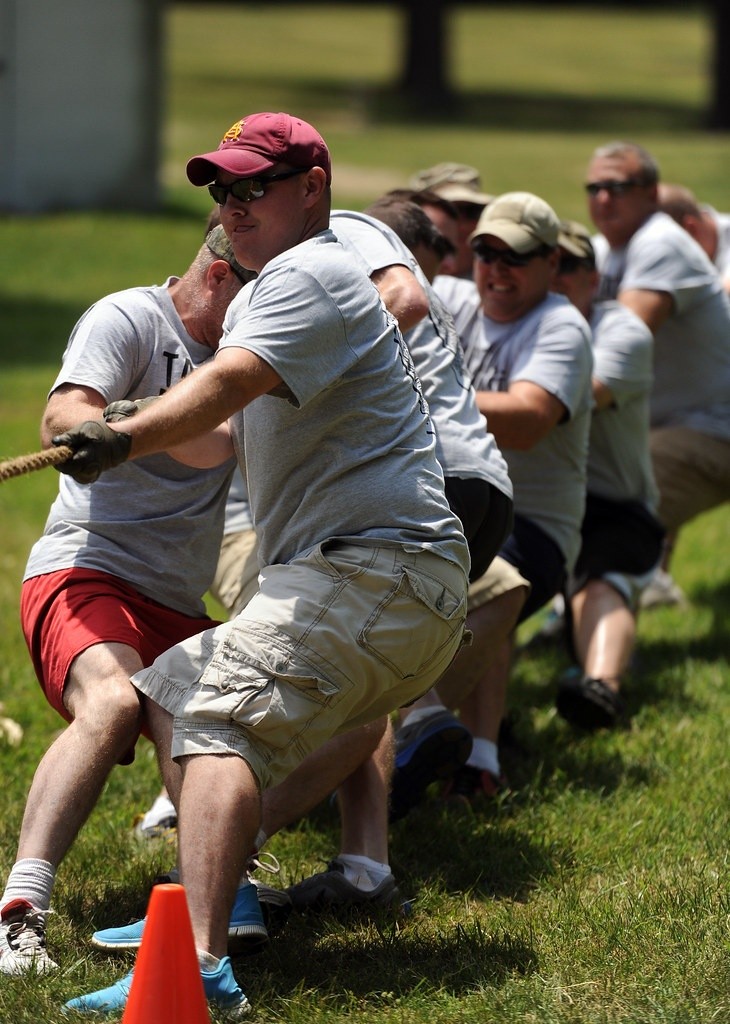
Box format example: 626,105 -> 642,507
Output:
555,666 -> 626,737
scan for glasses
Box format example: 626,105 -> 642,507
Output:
208,162 -> 312,208
558,256 -> 582,273
586,181 -> 649,199
474,241 -> 541,267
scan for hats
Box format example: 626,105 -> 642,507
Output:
558,220 -> 596,258
467,190 -> 562,254
412,162 -> 498,206
186,113 -> 331,189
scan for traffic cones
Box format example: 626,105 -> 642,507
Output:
121,883 -> 212,1024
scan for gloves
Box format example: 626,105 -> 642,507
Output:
52,421 -> 133,483
102,399 -> 138,425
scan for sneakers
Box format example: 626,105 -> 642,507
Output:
131,816 -> 179,849
62,954 -> 253,1022
90,883 -> 269,953
243,851 -> 293,933
279,861 -> 407,926
0,899 -> 63,977
328,709 -> 473,828
438,763 -> 503,813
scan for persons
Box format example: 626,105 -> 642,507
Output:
50,110 -> 472,1024
127,139 -> 729,931
0,222 -> 262,976
